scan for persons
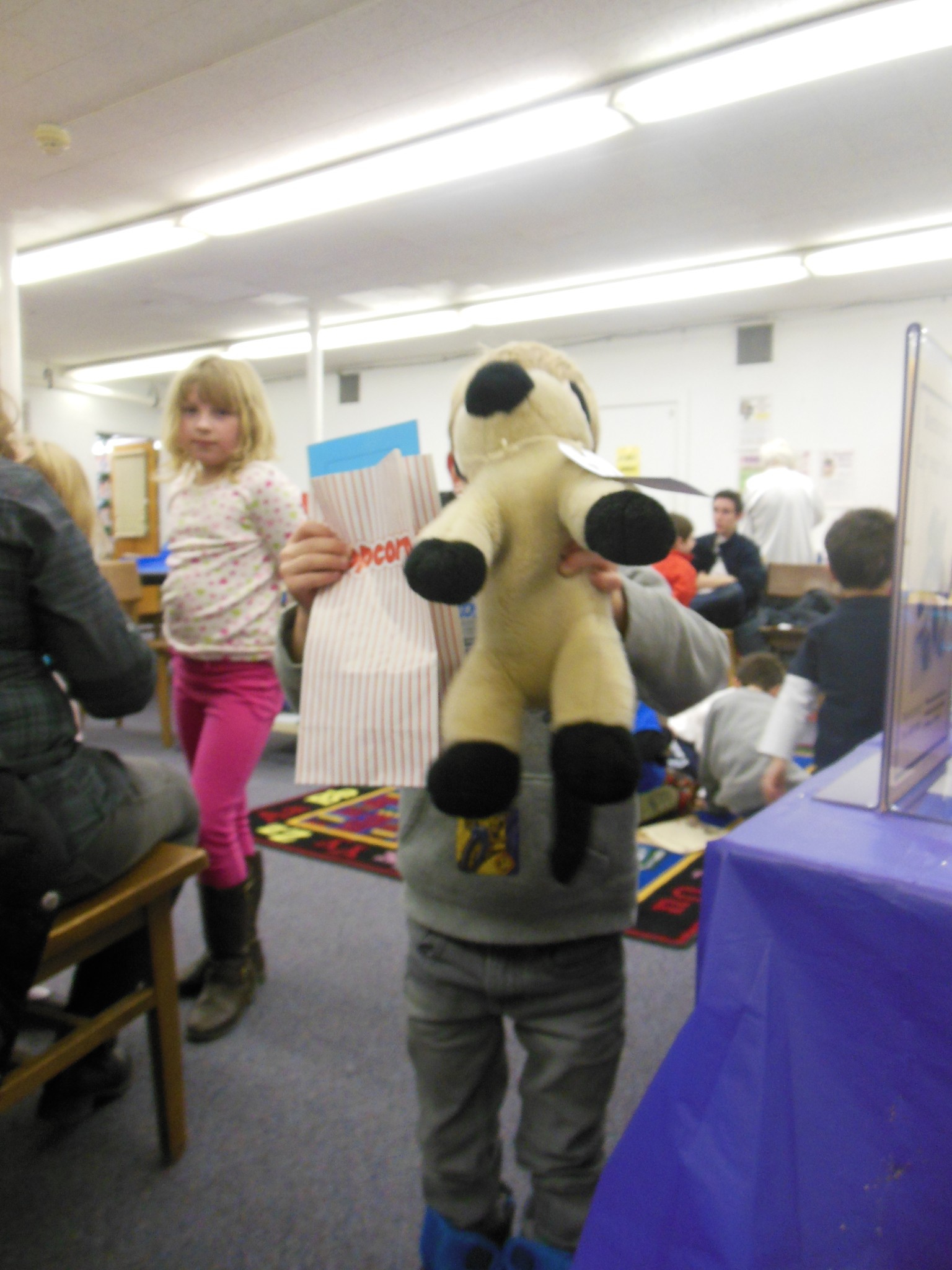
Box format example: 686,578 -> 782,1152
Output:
158,354 -> 307,1047
1,386 -> 203,1128
781,509 -> 895,774
684,489 -> 769,631
699,649 -> 814,825
275,337 -> 734,1270
651,508 -> 699,611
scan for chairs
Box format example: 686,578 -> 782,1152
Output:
0,835 -> 211,1168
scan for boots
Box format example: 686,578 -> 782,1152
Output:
178,850 -> 265,1042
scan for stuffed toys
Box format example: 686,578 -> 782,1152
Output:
398,361 -> 678,888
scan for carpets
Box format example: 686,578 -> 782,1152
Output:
247,739 -> 826,952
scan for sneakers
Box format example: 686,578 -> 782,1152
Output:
37,1045 -> 132,1128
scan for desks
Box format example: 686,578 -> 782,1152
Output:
573,720 -> 951,1268
